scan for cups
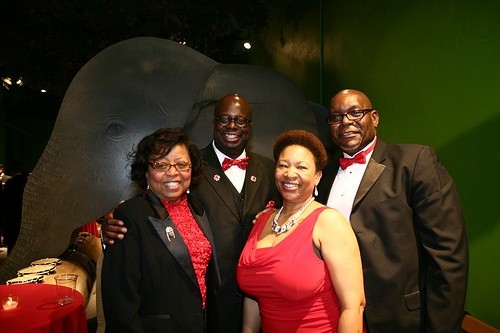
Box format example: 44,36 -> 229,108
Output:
54,273 -> 78,305
1,292 -> 18,310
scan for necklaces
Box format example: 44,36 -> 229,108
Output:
272,197 -> 315,235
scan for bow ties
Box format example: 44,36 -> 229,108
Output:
221,157 -> 250,172
338,136 -> 378,170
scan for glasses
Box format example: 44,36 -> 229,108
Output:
326,108 -> 376,124
214,114 -> 252,128
148,161 -> 193,172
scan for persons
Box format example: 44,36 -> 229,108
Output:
0,161 -> 30,258
101,93 -> 283,333
101,128 -> 222,333
253,89 -> 469,333
236,130 -> 366,333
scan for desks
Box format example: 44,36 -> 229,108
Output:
0,285 -> 87,333
